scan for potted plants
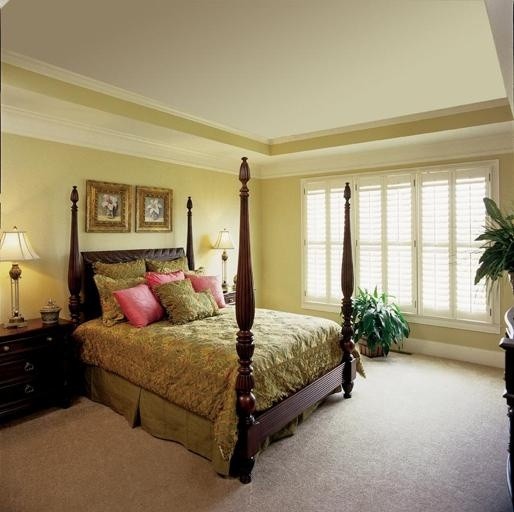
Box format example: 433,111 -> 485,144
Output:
338,286 -> 411,361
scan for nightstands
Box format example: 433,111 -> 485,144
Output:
1,315 -> 74,424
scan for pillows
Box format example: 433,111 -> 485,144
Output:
90,255 -> 227,329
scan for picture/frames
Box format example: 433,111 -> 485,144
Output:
85,180 -> 174,234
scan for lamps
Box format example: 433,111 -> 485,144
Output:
1,226 -> 41,329
210,227 -> 235,294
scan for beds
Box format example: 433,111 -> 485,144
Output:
67,156 -> 366,484
